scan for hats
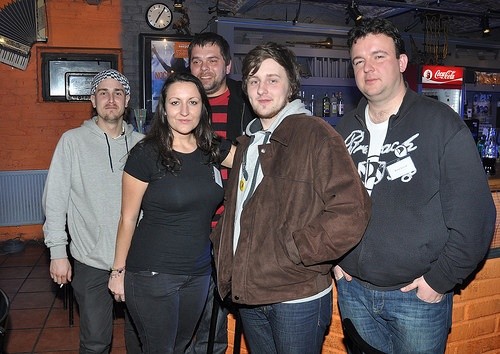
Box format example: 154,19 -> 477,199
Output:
90,70 -> 131,96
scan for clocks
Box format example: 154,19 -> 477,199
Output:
147,4 -> 172,31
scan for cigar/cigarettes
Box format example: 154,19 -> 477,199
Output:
60,284 -> 63,288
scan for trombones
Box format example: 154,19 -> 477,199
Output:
286,36 -> 350,49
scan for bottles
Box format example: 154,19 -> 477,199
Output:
337,92 -> 344,117
309,94 -> 316,116
330,93 -> 337,118
322,93 -> 330,117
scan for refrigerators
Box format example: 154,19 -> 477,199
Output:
404,63 -> 464,118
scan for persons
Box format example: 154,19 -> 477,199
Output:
329,17 -> 497,354
211,41 -> 372,354
42,70 -> 151,354
187,34 -> 255,354
106,73 -> 238,354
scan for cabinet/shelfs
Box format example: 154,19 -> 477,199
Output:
209,17 -> 364,129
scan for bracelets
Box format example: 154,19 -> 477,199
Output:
110,267 -> 126,279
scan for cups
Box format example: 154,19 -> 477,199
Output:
134,108 -> 147,134
